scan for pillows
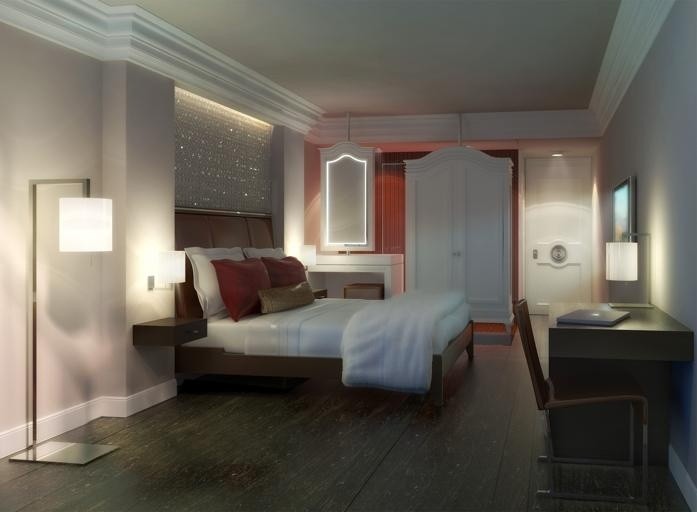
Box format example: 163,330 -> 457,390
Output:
183,246 -> 315,322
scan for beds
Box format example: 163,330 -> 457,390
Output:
175,206 -> 474,408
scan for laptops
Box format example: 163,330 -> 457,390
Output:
555,307 -> 631,326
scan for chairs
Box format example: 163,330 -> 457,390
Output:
513,299 -> 649,505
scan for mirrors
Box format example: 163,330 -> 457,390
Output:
317,141 -> 376,252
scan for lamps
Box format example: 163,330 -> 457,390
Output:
296,245 -> 316,271
8,178 -> 121,466
147,250 -> 187,291
605,233 -> 657,308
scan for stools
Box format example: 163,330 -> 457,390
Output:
343,282 -> 384,300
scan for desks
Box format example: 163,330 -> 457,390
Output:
549,302 -> 693,466
308,254 -> 405,300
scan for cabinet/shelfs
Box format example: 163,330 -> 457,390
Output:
402,146 -> 515,334
